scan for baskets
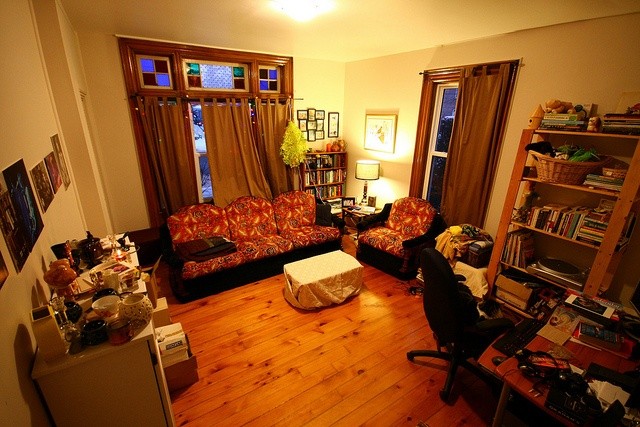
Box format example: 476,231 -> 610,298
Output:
529,150 -> 613,185
601,166 -> 627,180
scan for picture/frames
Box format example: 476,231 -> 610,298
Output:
329,112 -> 339,137
364,114 -> 398,154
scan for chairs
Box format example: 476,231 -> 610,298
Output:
406,248 -> 514,400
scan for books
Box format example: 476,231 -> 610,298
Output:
351,206 -> 376,216
564,294 -> 635,360
539,112 -> 588,131
154,322 -> 190,368
527,197 -> 637,252
583,173 -> 624,193
602,113 -> 640,134
305,169 -> 342,186
306,185 -> 341,199
501,228 -> 537,269
306,154 -> 341,169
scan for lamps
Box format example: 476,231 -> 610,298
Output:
297,108 -> 325,142
355,160 -> 380,203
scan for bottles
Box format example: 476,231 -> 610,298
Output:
326,142 -> 331,152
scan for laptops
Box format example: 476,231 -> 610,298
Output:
491,317 -> 548,357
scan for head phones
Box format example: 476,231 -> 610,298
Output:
514,347 -> 559,377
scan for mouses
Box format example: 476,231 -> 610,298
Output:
492,355 -> 507,365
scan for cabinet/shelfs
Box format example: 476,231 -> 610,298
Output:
33,235 -> 161,427
489,129 -> 640,317
298,152 -> 348,199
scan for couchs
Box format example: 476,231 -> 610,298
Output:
356,196 -> 442,282
163,191 -> 340,304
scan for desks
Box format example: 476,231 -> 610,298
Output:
475,303 -> 637,423
343,205 -> 383,222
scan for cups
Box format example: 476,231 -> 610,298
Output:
91,294 -> 121,319
83,319 -> 109,345
106,318 -> 134,345
